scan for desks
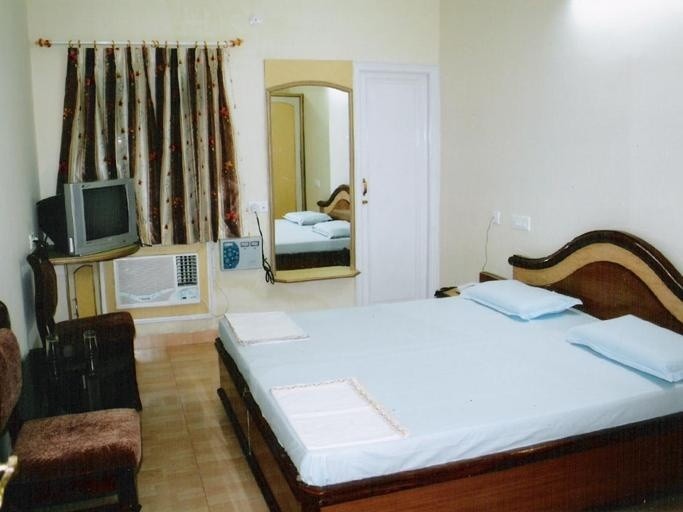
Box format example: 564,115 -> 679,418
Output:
48,242 -> 139,266
14,344 -> 140,426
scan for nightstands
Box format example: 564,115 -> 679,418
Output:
440,272 -> 504,297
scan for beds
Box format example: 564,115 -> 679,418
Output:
214,229 -> 683,511
274,184 -> 350,270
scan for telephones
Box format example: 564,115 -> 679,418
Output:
457,281 -> 475,293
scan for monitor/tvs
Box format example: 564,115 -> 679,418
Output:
36,177 -> 138,258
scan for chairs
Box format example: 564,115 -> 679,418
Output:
0,301 -> 142,512
27,246 -> 143,410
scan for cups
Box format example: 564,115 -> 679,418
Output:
82,330 -> 97,355
46,335 -> 60,361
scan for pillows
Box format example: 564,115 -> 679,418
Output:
569,313 -> 682,382
461,279 -> 583,320
316,219 -> 351,239
282,210 -> 333,223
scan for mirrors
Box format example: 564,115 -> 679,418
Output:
266,80 -> 360,282
